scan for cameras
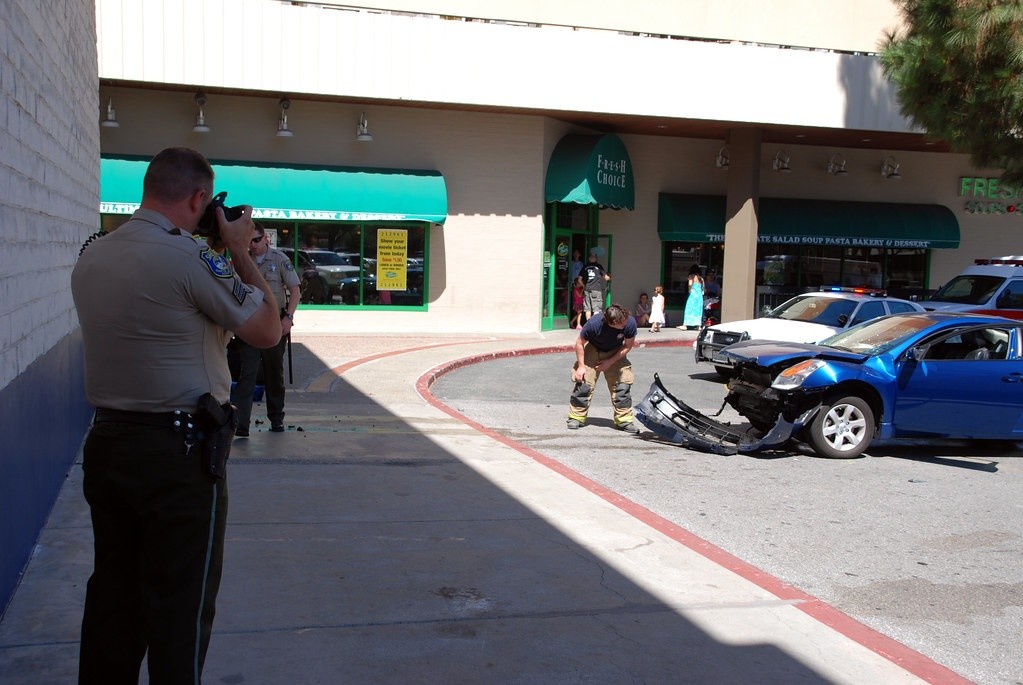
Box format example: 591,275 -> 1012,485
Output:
198,199 -> 242,243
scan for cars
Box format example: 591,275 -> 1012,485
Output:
338,267 -> 424,306
337,253 -> 424,269
692,286 -> 927,381
632,313 -> 1023,459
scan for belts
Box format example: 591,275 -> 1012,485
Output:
96,406 -> 200,431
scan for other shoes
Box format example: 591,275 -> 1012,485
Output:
677,325 -> 687,330
235,426 -> 248,435
567,419 -> 580,429
271,418 -> 283,430
570,321 -> 574,328
622,423 -> 639,432
576,325 -> 582,329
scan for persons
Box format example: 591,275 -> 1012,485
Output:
72,147 -> 282,685
566,303 -> 641,434
649,285 -> 666,333
635,293 -> 651,327
234,221 -> 301,438
677,264 -> 722,331
301,269 -> 323,304
543,248 -> 612,330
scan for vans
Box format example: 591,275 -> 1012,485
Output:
914,259 -> 1023,312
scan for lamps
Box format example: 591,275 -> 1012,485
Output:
275,95 -> 293,137
772,149 -> 792,173
193,89 -> 211,132
828,152 -> 848,175
102,96 -> 119,128
881,155 -> 902,180
356,111 -> 373,141
714,144 -> 730,170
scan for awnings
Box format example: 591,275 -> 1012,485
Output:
545,133 -> 635,211
100,153 -> 448,226
657,192 -> 961,249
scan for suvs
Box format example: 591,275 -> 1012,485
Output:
281,249 -> 367,304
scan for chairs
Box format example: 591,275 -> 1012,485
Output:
944,327 -> 991,360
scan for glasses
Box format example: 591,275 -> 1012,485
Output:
250,235 -> 264,244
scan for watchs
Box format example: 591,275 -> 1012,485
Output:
286,314 -> 293,320
